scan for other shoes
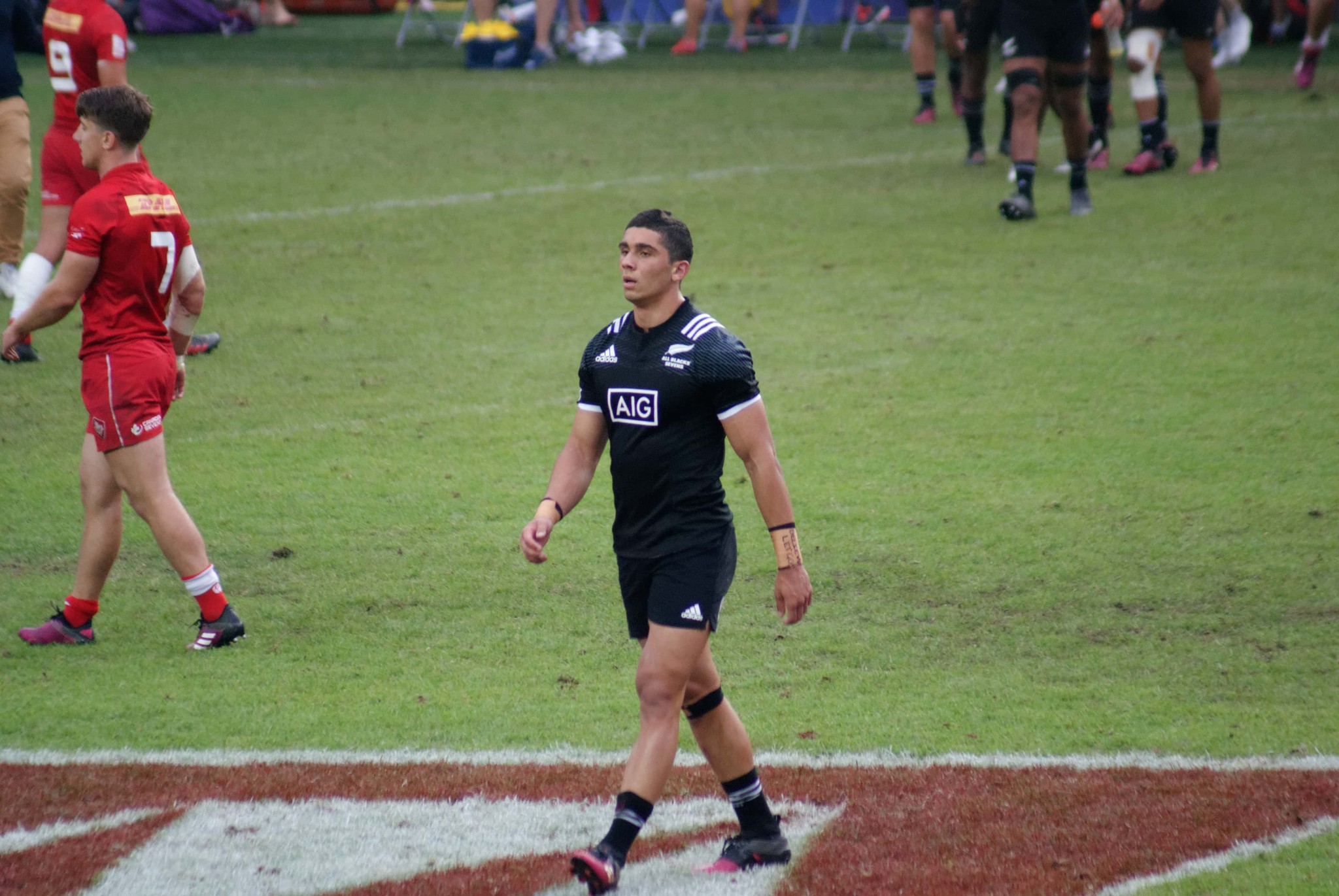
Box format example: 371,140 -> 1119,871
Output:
1294,42 -> 1322,89
670,40 -> 696,55
722,35 -> 748,51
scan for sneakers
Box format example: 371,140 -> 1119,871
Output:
953,96 -> 964,115
1082,144 -> 1110,170
1189,151 -> 1220,174
691,833 -> 790,873
999,195 -> 1035,220
185,602 -> 245,650
569,845 -> 621,896
1068,183 -> 1092,217
0,339 -> 38,363
966,144 -> 987,166
1125,140 -> 1178,174
17,604 -> 94,647
913,104 -> 936,124
185,332 -> 219,355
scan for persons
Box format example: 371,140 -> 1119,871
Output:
0,0 -> 220,362
521,209 -> 811,896
0,85 -> 247,649
460,0 -> 1339,221
